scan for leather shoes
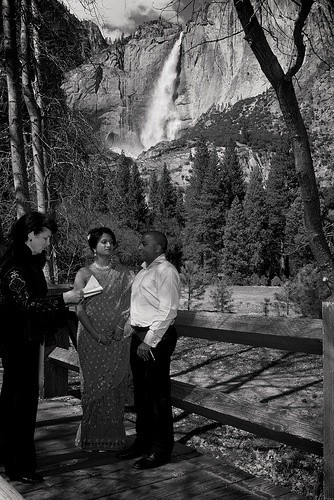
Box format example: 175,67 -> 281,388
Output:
10,471 -> 43,484
133,451 -> 165,470
116,446 -> 139,460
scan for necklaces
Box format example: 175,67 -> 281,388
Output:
93,258 -> 115,272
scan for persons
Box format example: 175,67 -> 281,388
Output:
117,230 -> 180,470
74,227 -> 132,455
0,211 -> 86,484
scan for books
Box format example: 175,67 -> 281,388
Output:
78,275 -> 104,298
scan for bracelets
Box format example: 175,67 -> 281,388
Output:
115,323 -> 124,331
98,334 -> 101,343
